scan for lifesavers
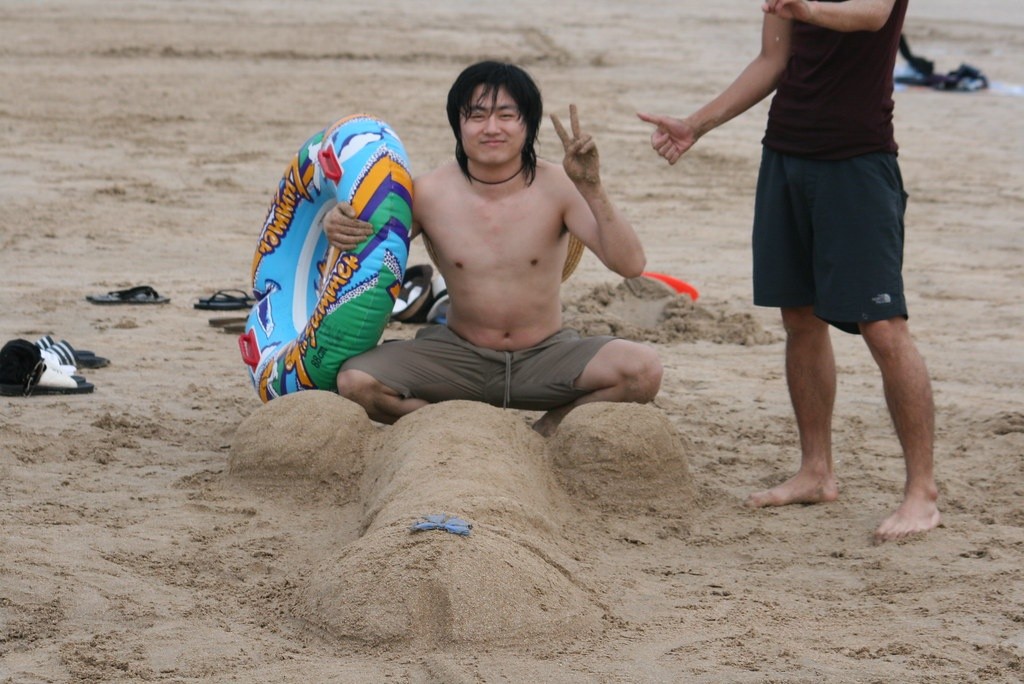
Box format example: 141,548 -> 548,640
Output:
236,113 -> 414,406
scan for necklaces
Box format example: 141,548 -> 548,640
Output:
468,163 -> 524,184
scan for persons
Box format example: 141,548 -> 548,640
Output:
323,57 -> 665,439
636,0 -> 940,546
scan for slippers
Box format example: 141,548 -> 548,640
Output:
0,365 -> 96,395
83,284 -> 255,333
22,337 -> 116,371
